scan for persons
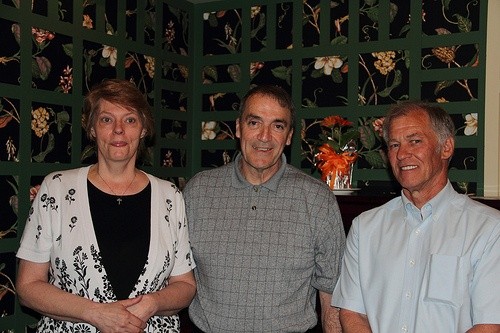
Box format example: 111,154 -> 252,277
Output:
183,83 -> 347,333
15,79 -> 197,333
330,100 -> 500,333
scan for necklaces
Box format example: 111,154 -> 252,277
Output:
96,168 -> 138,204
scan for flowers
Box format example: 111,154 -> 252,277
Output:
318,115 -> 352,156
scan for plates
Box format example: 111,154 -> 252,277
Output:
332,188 -> 362,195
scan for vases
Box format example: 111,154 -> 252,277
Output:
316,153 -> 354,190
